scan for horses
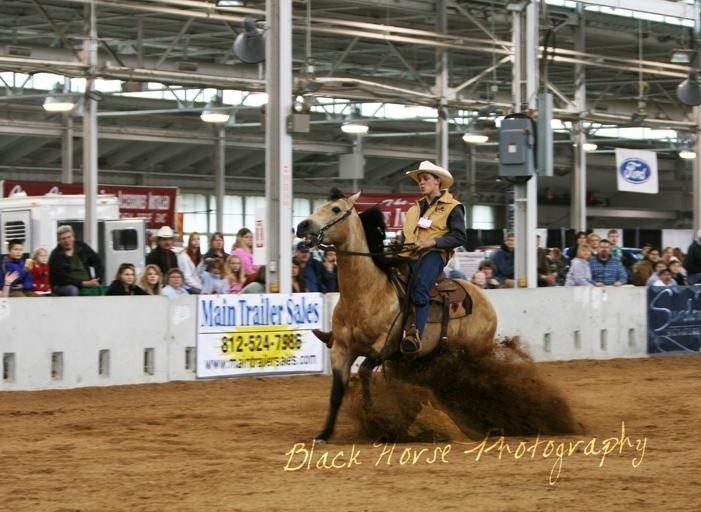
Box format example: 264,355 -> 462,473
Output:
297,186 -> 500,448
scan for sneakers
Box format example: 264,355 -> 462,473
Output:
312,329 -> 327,343
402,336 -> 418,361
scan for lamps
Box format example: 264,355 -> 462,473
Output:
42,83 -> 76,112
340,108 -> 370,134
678,141 -> 696,159
463,117 -> 490,146
200,95 -> 230,124
573,134 -> 598,152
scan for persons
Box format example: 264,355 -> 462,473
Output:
445,225 -> 701,288
1,223 -> 337,295
391,160 -> 467,355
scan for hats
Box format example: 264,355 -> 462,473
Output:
405,161 -> 455,189
296,242 -> 309,251
151,226 -> 178,238
